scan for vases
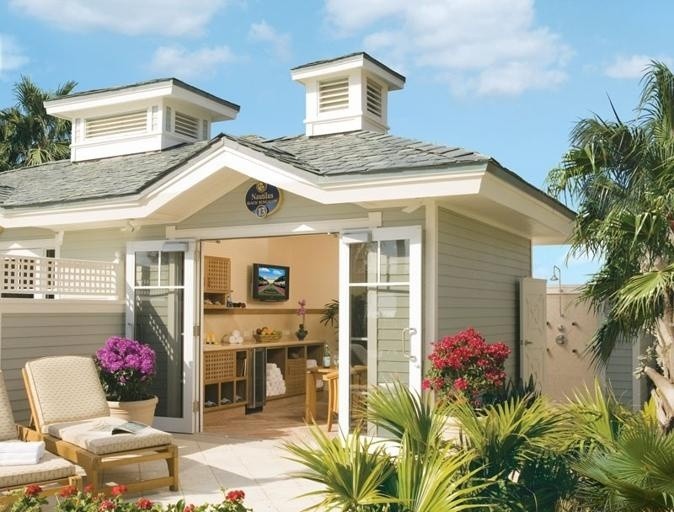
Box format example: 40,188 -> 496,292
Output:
104,394 -> 158,428
433,410 -> 487,456
297,322 -> 307,341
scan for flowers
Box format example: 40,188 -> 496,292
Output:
296,299 -> 306,323
424,327 -> 512,411
95,335 -> 155,401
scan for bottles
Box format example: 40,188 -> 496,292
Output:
322,344 -> 330,368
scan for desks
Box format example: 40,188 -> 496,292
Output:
301,365 -> 334,426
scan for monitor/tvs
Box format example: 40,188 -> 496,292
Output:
251,263 -> 289,301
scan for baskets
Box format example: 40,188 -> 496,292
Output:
253,331 -> 282,344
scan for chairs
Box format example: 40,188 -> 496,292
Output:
0,354 -> 183,505
322,365 -> 367,432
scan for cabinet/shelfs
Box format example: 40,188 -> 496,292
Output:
202,339 -> 325,421
201,256 -> 233,309
